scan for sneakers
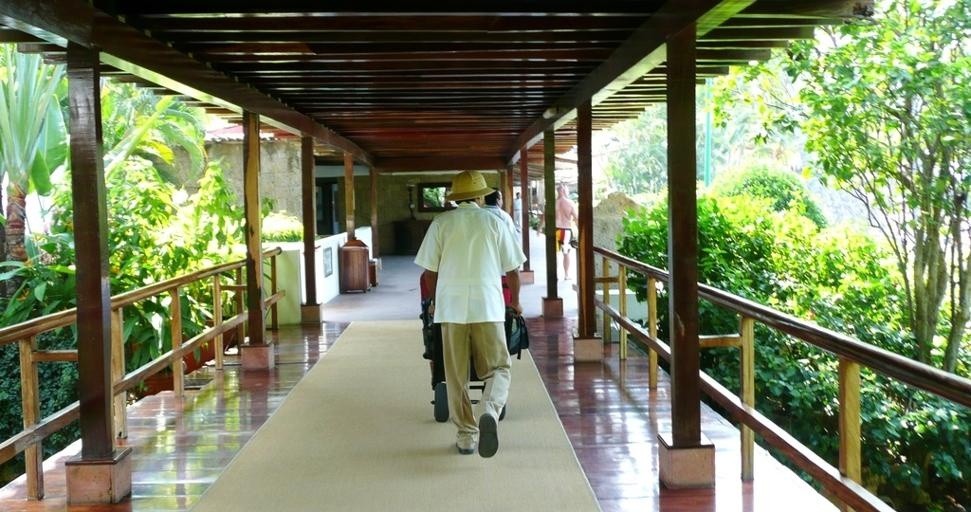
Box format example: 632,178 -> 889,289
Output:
478,410 -> 499,458
454,427 -> 479,455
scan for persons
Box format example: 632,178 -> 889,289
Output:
482,184 -> 519,242
410,167 -> 525,459
554,184 -> 580,281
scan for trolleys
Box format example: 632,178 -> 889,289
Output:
421,295 -> 520,421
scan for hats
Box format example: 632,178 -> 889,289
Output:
443,171 -> 497,203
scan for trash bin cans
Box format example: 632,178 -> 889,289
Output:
339,240 -> 370,293
